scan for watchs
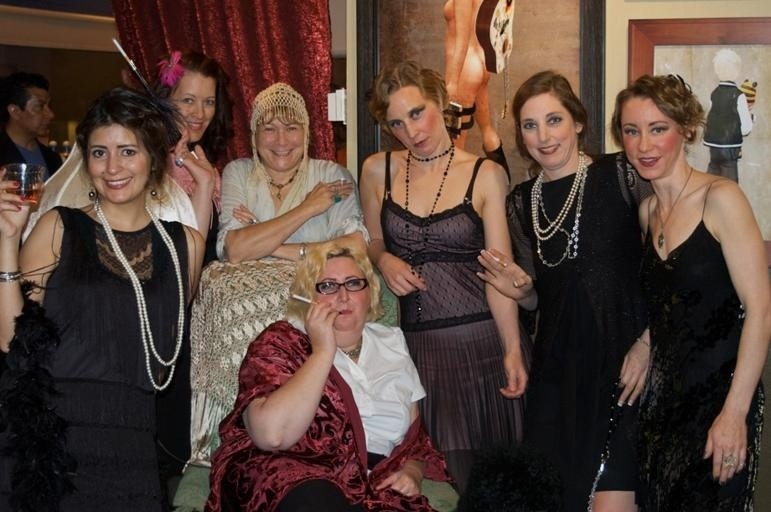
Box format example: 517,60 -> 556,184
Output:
173,149 -> 189,168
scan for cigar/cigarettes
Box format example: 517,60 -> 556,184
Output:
485,251 -> 509,267
291,293 -> 320,305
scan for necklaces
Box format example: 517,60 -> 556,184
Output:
342,345 -> 361,361
268,171 -> 296,200
402,140 -> 457,324
94,198 -> 186,393
529,151 -> 590,269
652,167 -> 693,249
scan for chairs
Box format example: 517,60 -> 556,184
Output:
174,258 -> 458,512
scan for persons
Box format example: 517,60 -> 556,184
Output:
0,74 -> 62,182
215,80 -> 368,266
0,86 -> 209,512
438,1 -> 516,183
142,46 -> 221,267
609,70 -> 770,512
202,232 -> 453,512
502,68 -> 658,512
697,47 -> 756,188
355,57 -> 535,497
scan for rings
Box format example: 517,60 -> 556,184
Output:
248,218 -> 256,224
723,455 -> 735,466
333,194 -> 341,203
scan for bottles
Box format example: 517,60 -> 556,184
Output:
47,140 -> 72,158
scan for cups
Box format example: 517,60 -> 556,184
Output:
6,163 -> 44,205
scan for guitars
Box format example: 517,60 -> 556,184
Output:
475,0 -> 513,118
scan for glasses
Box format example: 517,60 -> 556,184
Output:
314,276 -> 368,296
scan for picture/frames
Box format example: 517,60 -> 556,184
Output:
629,16 -> 771,266
356,0 -> 606,193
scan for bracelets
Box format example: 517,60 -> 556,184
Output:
0,270 -> 21,283
298,241 -> 308,262
634,337 -> 651,350
